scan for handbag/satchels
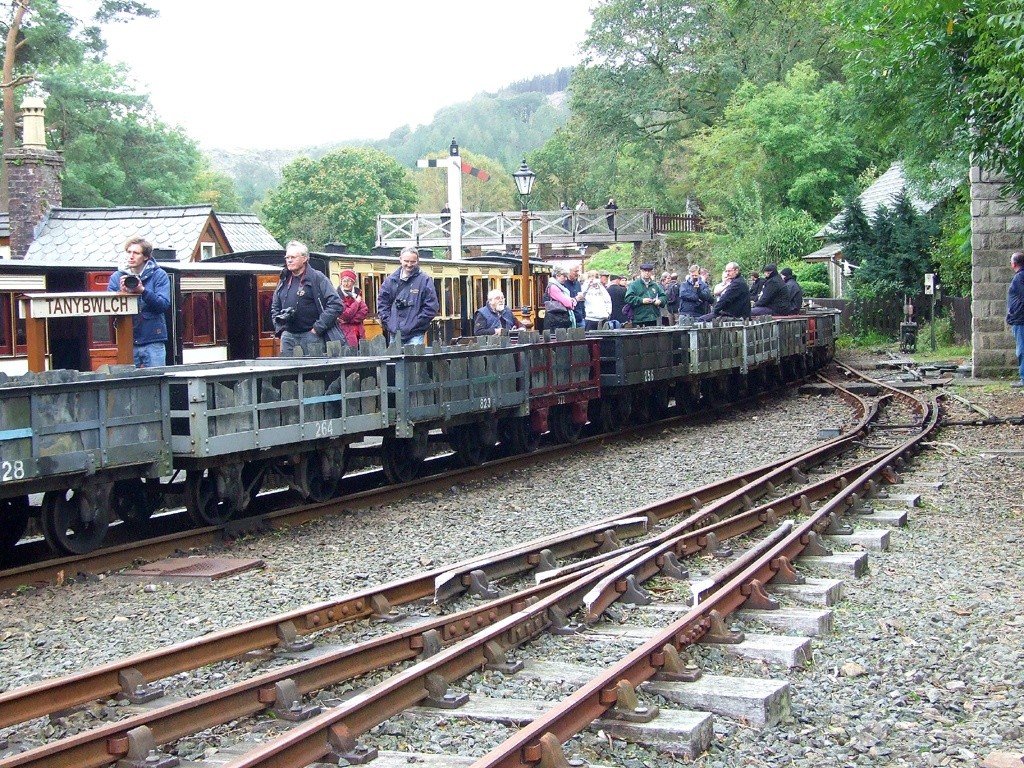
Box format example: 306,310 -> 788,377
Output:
622,304 -> 634,321
544,284 -> 572,329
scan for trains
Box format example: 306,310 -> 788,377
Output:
0,303 -> 840,573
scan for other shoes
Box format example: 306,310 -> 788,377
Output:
1010,381 -> 1024,388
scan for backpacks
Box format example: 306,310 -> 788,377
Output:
667,283 -> 678,304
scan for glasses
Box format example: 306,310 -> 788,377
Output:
284,255 -> 304,260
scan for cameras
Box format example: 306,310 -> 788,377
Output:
274,306 -> 296,326
124,274 -> 141,290
395,298 -> 416,309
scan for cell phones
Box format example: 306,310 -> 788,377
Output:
693,278 -> 698,285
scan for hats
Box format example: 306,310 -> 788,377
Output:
764,264 -> 777,271
639,264 -> 653,270
781,268 -> 792,276
610,275 -> 620,281
340,271 -> 357,283
599,269 -> 609,276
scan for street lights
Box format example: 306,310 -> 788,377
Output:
513,160 -> 537,328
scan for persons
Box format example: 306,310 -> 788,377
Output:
545,259 -> 802,338
560,199 -> 590,231
271,241 -> 348,357
377,246 -> 439,345
441,202 -> 466,237
1005,252 -> 1024,387
604,199 -> 618,233
473,289 -> 523,336
107,237 -> 171,367
336,269 -> 369,345
